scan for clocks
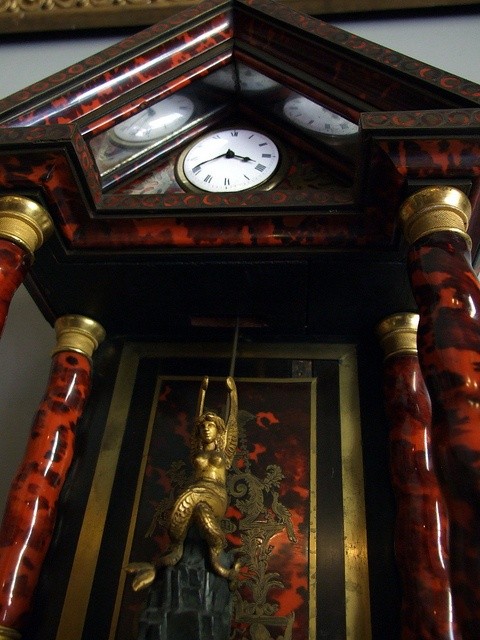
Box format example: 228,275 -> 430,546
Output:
277,87 -> 366,148
173,123 -> 291,194
106,89 -> 201,148
1,1 -> 480,640
206,60 -> 279,96
